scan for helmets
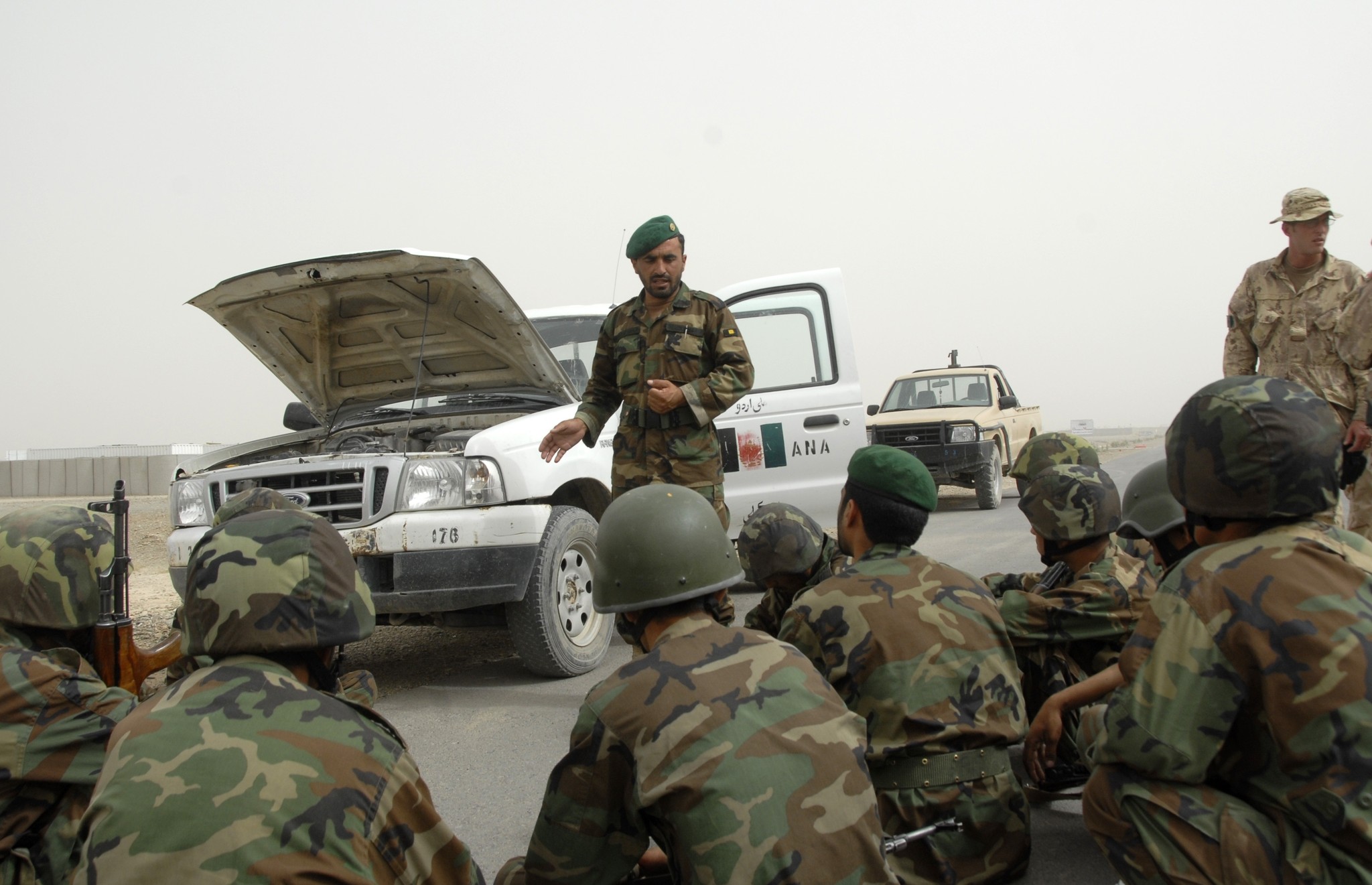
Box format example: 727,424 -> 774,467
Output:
1018,463 -> 1121,542
1117,456 -> 1185,540
1008,431 -> 1100,477
0,505 -> 135,627
593,484 -> 746,613
178,507 -> 376,657
212,487 -> 303,528
740,502 -> 823,585
1166,375 -> 1346,522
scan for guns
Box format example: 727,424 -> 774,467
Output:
885,817 -> 964,854
88,480 -> 185,696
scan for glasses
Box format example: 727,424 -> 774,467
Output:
1301,216 -> 1336,228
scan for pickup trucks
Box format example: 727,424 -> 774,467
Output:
866,349 -> 1042,511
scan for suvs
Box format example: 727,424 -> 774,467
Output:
165,247 -> 869,685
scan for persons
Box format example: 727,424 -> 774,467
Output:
1221,186 -> 1371,539
538,214 -> 765,660
979,374 -> 1372,885
0,486 -> 488,885
491,443 -> 1033,885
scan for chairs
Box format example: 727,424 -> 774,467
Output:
560,359 -> 590,385
917,391 -> 937,406
962,382 -> 988,401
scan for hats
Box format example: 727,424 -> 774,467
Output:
1269,188 -> 1344,225
626,215 -> 679,259
847,444 -> 939,516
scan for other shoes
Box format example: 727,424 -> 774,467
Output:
1040,763 -> 1091,791
